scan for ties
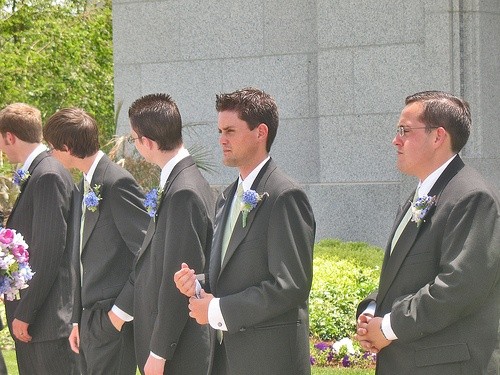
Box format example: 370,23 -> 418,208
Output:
390,186 -> 420,256
220,182 -> 244,268
80,178 -> 89,240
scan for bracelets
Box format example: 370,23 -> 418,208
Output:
380,322 -> 388,340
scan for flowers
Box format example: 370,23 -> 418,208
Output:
144,186 -> 164,223
11,169 -> 32,194
0,226 -> 36,301
408,196 -> 438,228
238,188 -> 270,229
83,184 -> 102,213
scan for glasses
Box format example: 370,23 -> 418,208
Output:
127,135 -> 155,145
46,145 -> 60,155
397,126 -> 447,136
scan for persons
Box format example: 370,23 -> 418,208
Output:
0,103 -> 76,375
174,87 -> 317,375
42,106 -> 154,375
135,94 -> 216,375
354,91 -> 500,375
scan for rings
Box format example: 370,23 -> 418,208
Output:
20,333 -> 22,335
